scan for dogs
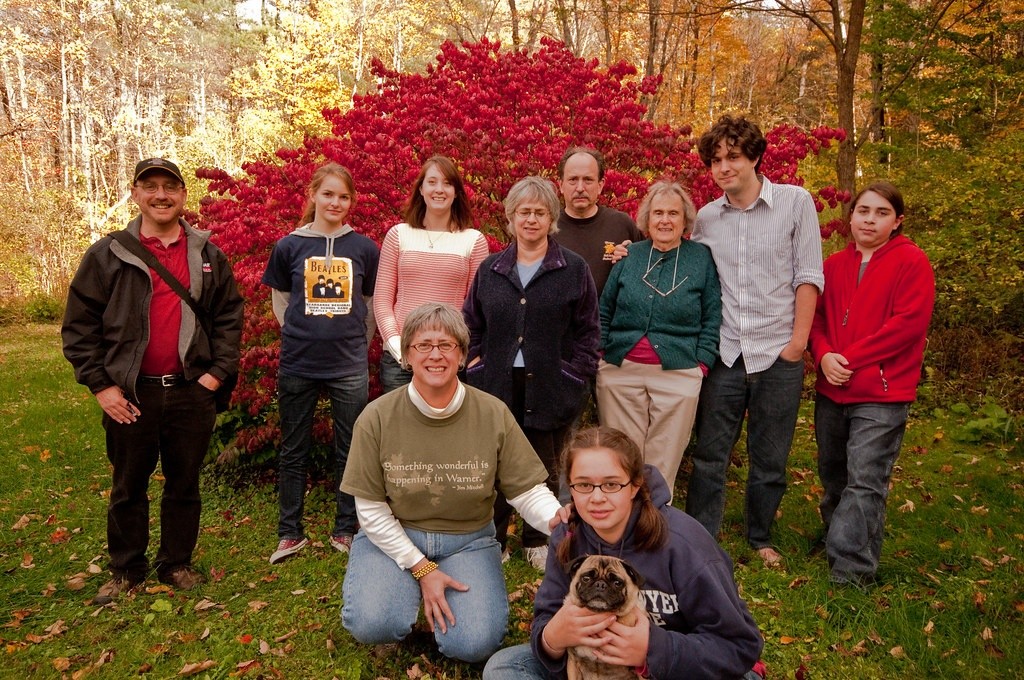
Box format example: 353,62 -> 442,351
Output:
562,553 -> 647,680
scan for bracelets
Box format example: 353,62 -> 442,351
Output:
541,627 -> 566,654
411,561 -> 441,582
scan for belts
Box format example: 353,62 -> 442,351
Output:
138,374 -> 188,387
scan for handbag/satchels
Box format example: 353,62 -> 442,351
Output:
217,370 -> 236,413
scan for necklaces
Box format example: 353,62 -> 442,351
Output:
420,220 -> 447,248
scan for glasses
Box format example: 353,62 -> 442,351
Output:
515,207 -> 551,218
567,478 -> 635,492
135,181 -> 183,192
409,341 -> 461,353
642,258 -> 688,297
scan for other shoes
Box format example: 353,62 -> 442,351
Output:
807,540 -> 836,567
757,547 -> 784,568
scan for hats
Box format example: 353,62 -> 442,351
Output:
134,157 -> 185,188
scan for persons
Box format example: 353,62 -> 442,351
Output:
549,148 -> 646,303
461,175 -> 601,575
261,164 -> 381,565
611,115 -> 825,567
481,428 -> 765,680
595,182 -> 723,506
339,302 -> 573,663
373,156 -> 490,395
806,183 -> 935,591
61,159 -> 245,599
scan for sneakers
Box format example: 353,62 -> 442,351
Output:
92,562 -> 149,605
157,564 -> 207,591
329,533 -> 353,555
501,548 -> 511,580
270,537 -> 308,565
522,544 -> 550,576
